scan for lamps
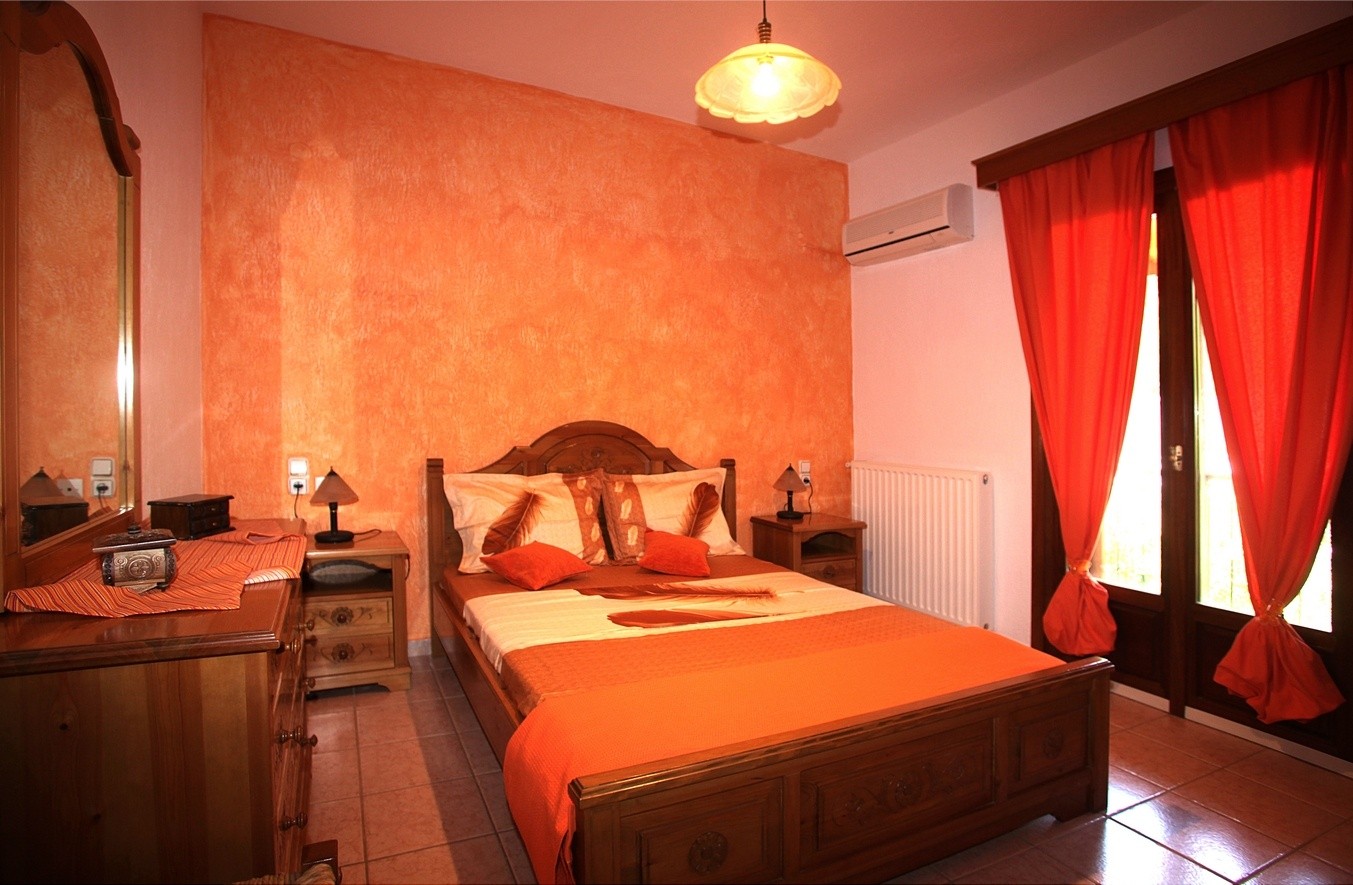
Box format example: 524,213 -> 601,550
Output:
773,463 -> 809,520
689,1 -> 843,132
308,468 -> 359,543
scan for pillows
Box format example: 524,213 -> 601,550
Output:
594,468 -> 746,564
440,468 -> 614,573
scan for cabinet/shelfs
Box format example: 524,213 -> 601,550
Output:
4,515 -> 345,883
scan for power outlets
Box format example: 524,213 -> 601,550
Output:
799,473 -> 812,487
287,476 -> 312,497
89,474 -> 115,497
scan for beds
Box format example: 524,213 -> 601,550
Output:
426,422 -> 1113,883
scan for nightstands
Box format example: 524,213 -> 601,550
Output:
751,510 -> 869,594
298,529 -> 416,699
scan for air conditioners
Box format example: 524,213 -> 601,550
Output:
840,181 -> 980,268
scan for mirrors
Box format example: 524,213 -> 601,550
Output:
0,0 -> 152,592
